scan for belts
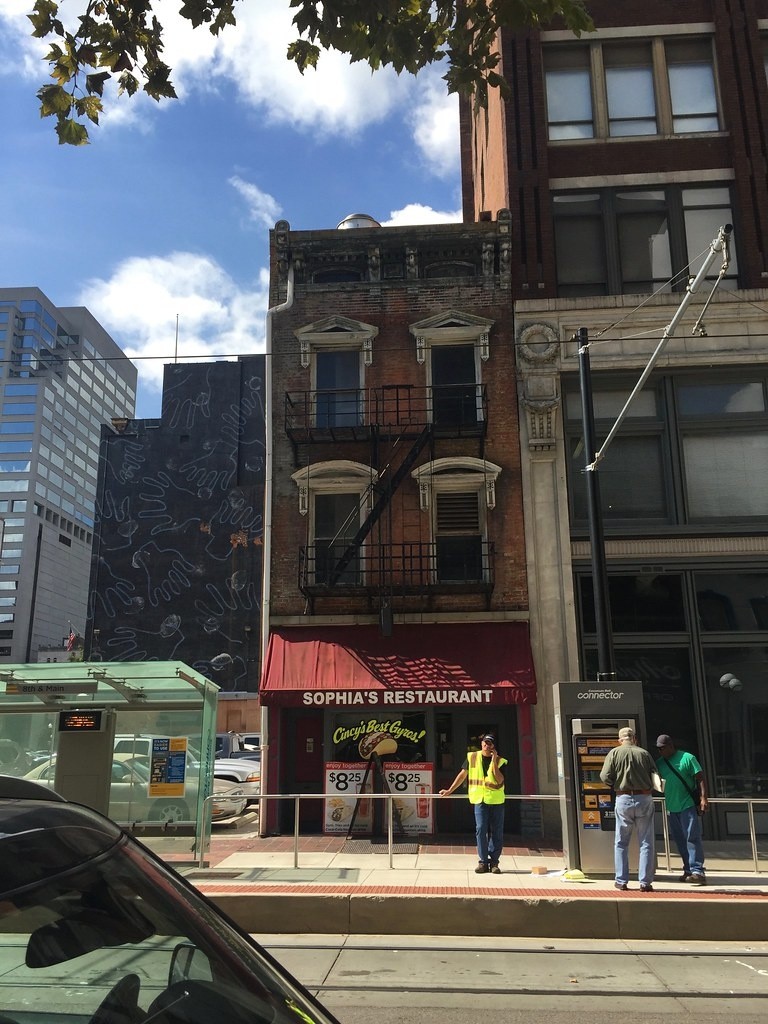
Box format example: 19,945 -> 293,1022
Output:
615,790 -> 652,794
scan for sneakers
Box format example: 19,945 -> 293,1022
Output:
490,863 -> 500,874
475,862 -> 489,873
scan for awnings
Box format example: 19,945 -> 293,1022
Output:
258,630 -> 537,706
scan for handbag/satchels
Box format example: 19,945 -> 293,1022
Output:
691,788 -> 701,806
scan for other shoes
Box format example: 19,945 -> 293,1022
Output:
685,873 -> 706,883
614,882 -> 627,890
640,885 -> 653,892
679,872 -> 693,882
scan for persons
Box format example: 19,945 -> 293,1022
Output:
439,735 -> 508,874
599,727 -> 662,892
654,735 -> 709,885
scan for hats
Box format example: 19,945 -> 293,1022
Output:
656,734 -> 672,747
618,727 -> 635,738
483,733 -> 497,746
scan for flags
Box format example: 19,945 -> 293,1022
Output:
67,627 -> 75,651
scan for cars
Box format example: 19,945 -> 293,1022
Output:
1,720 -> 343,1023
